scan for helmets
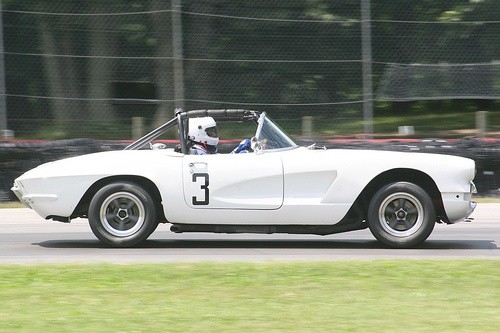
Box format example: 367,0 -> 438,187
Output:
187,115 -> 220,145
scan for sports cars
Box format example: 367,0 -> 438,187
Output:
10,107 -> 478,249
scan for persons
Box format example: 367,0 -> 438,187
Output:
186,116 -> 251,154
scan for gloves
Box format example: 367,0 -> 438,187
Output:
234,139 -> 250,153
